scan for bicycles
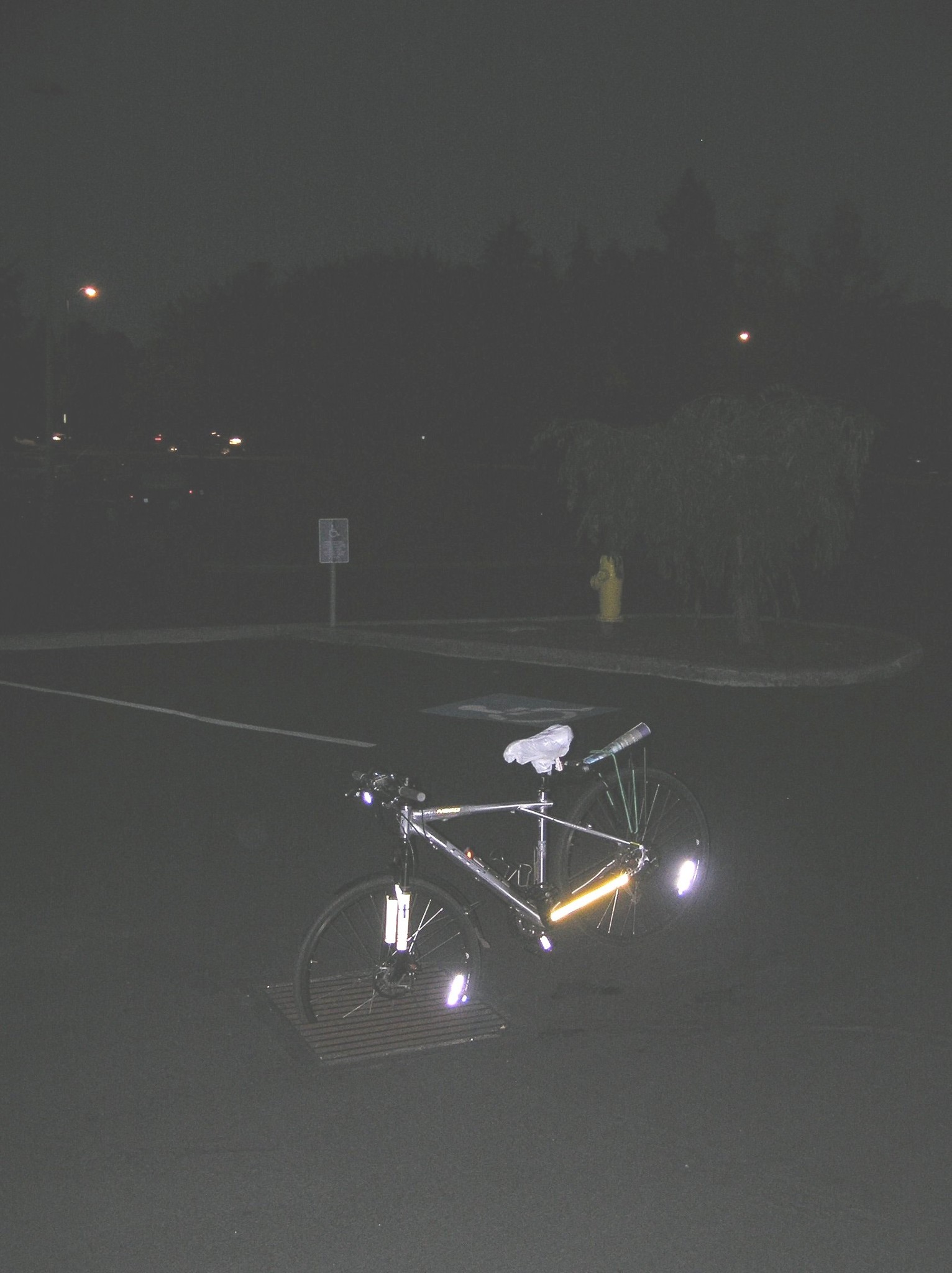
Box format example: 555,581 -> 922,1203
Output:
295,719 -> 712,1026
